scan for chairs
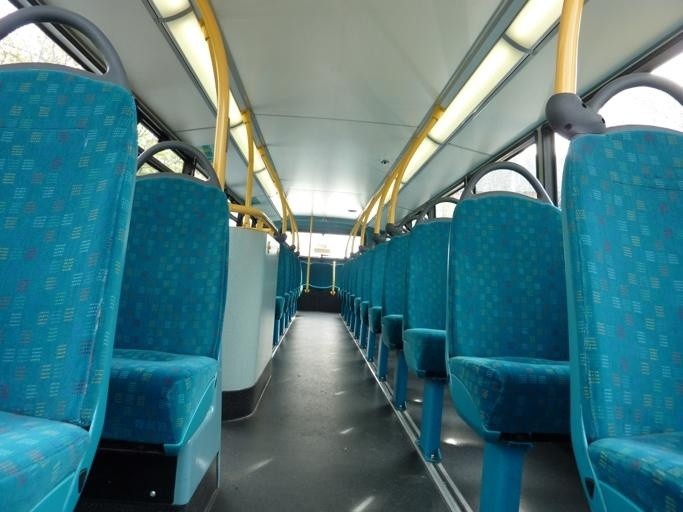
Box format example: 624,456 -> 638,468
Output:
300,257 -> 337,294
